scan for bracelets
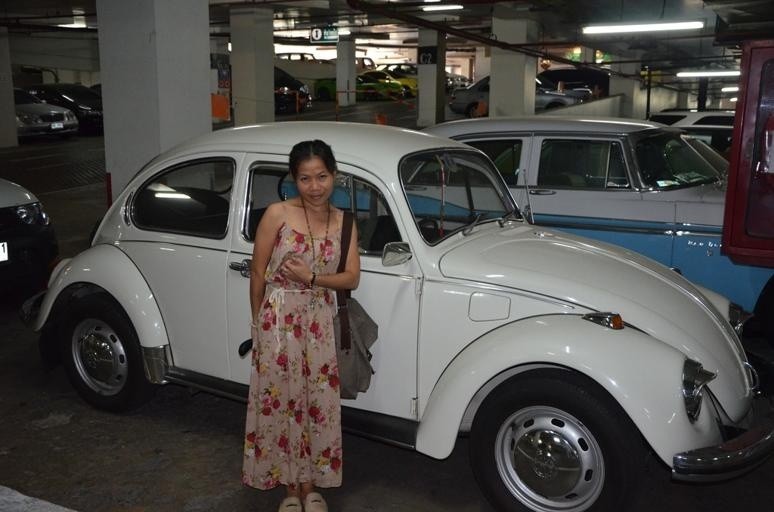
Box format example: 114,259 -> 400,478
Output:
306,269 -> 317,288
248,321 -> 259,332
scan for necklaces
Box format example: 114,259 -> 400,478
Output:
298,195 -> 332,265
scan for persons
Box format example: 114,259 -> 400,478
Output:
241,139 -> 379,512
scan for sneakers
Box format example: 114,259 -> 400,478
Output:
278,497 -> 303,511
304,493 -> 328,512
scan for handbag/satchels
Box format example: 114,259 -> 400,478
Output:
334,299 -> 378,401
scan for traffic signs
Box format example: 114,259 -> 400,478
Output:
310,26 -> 339,42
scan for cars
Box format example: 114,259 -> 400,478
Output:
13,83 -> 103,142
447,73 -> 585,118
648,105 -> 771,174
0,175 -> 63,308
209,48 -> 472,117
31,118 -> 774,510
538,65 -> 610,100
278,116 -> 774,341
